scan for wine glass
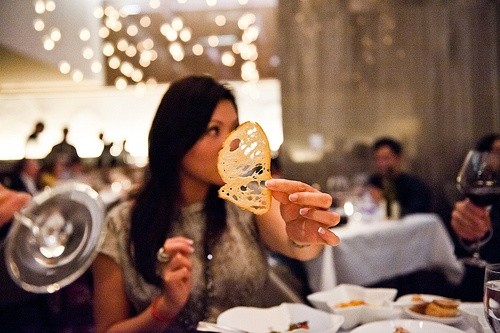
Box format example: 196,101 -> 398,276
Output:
483,264 -> 500,333
457,148 -> 500,266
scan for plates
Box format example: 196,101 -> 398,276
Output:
348,319 -> 466,333
396,292 -> 463,322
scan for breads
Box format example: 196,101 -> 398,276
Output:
217,120 -> 273,215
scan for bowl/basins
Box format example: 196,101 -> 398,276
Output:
307,283 -> 398,330
216,304 -> 343,333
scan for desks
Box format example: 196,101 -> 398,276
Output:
306,213 -> 463,292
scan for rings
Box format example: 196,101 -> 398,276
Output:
156,247 -> 169,264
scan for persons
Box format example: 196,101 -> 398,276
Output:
360,136 -> 433,220
451,132 -> 500,271
89,76 -> 341,333
22,120 -> 132,176
